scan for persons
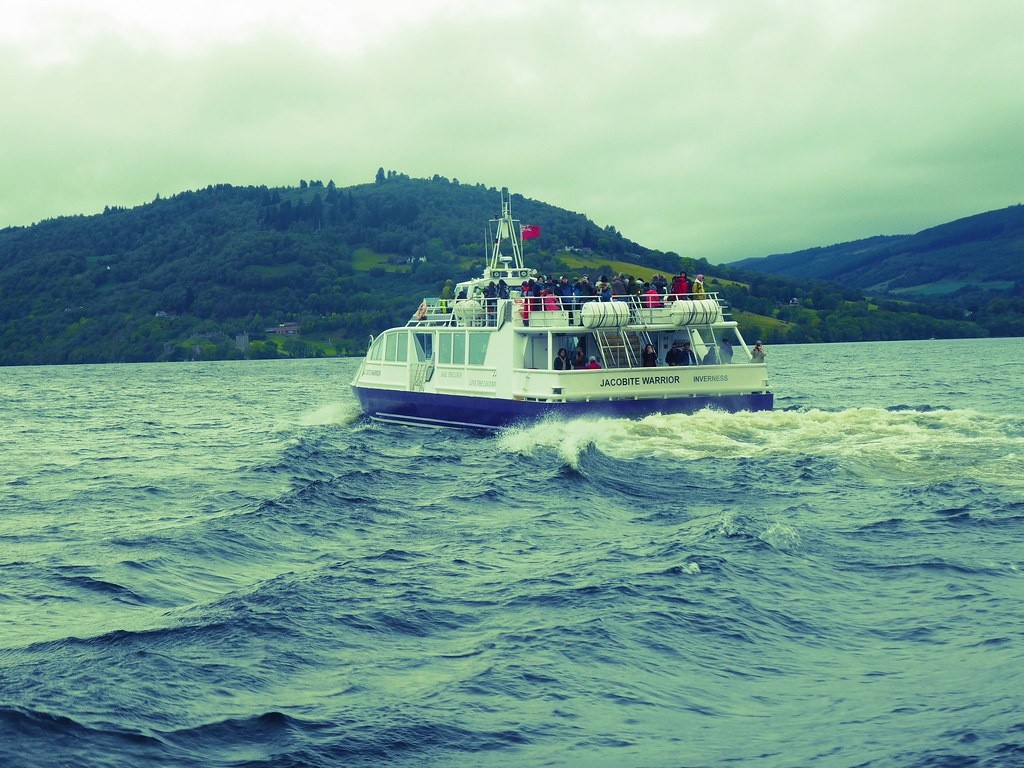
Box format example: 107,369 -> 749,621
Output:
553,348 -> 571,370
703,347 -> 720,365
586,356 -> 601,369
571,347 -> 589,369
442,271 -> 706,327
680,343 -> 697,365
642,344 -> 657,367
720,338 -> 733,364
750,340 -> 766,363
666,343 -> 682,366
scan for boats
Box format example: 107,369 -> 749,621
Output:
348,182 -> 775,433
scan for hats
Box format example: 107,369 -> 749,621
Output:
697,275 -> 704,281
601,276 -> 607,282
574,277 -> 578,279
563,276 -> 568,279
590,356 -> 595,361
680,270 -> 686,275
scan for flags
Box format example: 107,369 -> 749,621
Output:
520,224 -> 539,240
